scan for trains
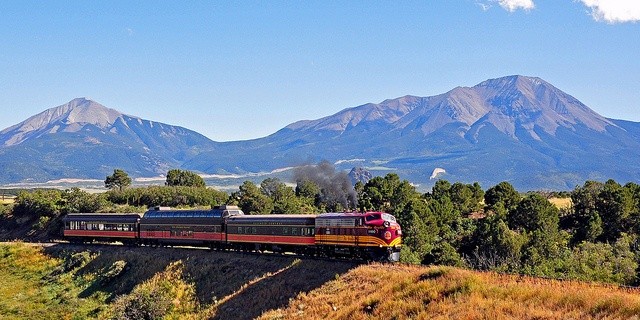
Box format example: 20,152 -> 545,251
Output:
59,205 -> 402,262
